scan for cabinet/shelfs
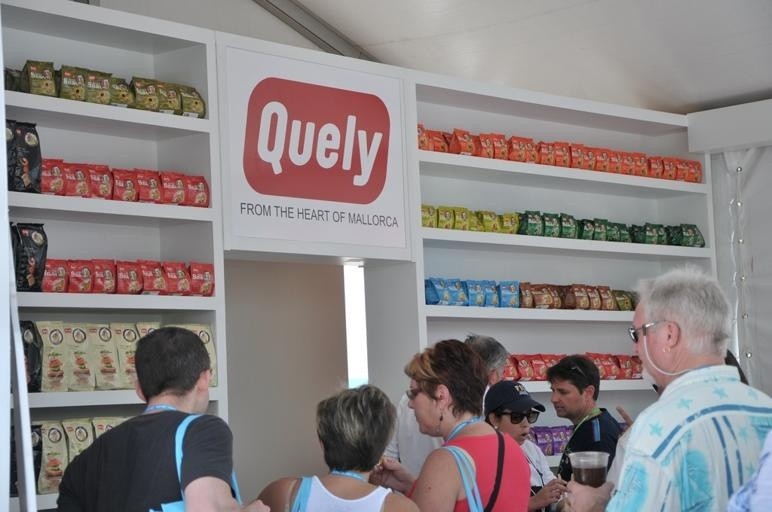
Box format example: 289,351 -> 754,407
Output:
1,1 -> 228,512
365,74 -> 723,468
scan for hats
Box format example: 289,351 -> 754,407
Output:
485,381 -> 545,416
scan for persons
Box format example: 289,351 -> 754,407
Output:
483,381 -> 571,512
381,334 -> 507,480
728,430 -> 771,511
56,326 -> 272,511
257,385 -> 420,512
605,347 -> 749,494
545,354 -> 624,485
603,269 -> 772,512
368,339 -> 531,512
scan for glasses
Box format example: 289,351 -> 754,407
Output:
561,357 -> 585,378
629,324 -> 655,343
406,390 -> 421,400
502,412 -> 539,423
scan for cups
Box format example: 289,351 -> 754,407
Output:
567,450 -> 610,491
